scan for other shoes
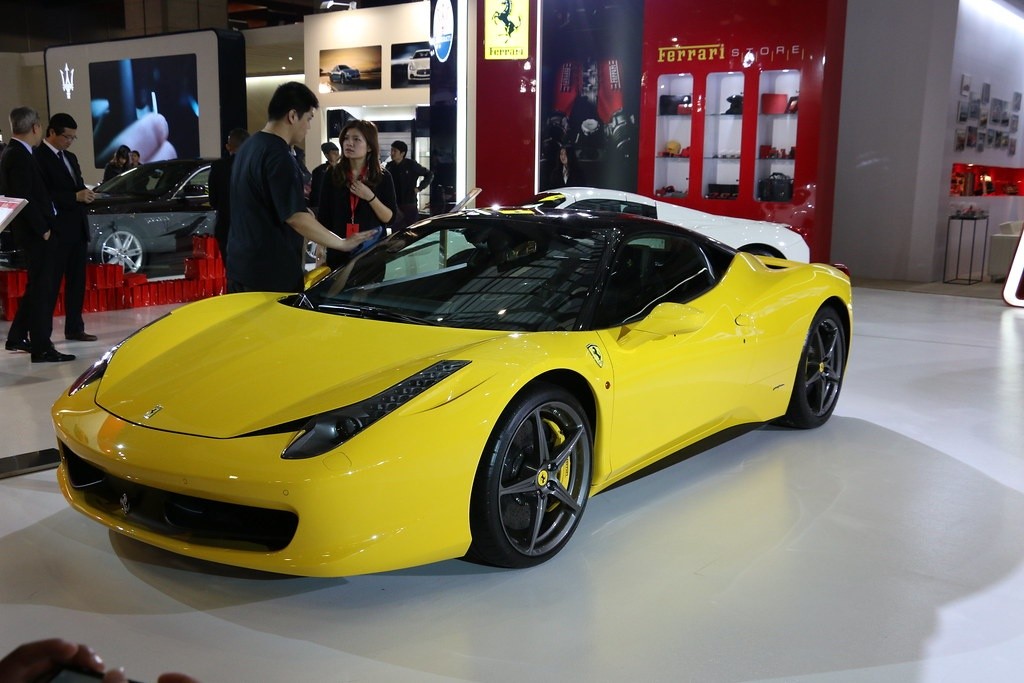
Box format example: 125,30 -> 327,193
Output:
65,331 -> 97,341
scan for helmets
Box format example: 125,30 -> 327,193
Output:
665,141 -> 681,154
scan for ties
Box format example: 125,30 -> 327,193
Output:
57,151 -> 63,160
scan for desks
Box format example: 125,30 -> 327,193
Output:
942,215 -> 989,285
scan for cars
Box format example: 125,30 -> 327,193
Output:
407,49 -> 430,81
86,158 -> 223,270
330,65 -> 360,85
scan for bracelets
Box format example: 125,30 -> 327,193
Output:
369,194 -> 376,202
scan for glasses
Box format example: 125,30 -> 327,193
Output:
62,133 -> 79,142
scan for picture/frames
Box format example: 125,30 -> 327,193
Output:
953,74 -> 1022,155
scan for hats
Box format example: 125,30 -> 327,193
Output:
321,142 -> 339,154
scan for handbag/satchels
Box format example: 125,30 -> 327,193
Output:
761,173 -> 792,202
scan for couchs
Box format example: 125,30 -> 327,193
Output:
990,221 -> 1024,282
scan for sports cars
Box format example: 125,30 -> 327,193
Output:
49,206 -> 853,580
518,187 -> 810,266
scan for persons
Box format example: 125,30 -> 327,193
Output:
226,82 -> 377,293
0,106 -> 143,362
94,105 -> 177,166
318,119 -> 401,271
210,128 -> 435,268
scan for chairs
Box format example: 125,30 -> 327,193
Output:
444,244 -> 655,314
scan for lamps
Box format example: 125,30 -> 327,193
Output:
320,0 -> 357,12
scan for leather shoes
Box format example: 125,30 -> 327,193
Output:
31,346 -> 76,362
6,337 -> 31,354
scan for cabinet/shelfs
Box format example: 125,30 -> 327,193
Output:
655,66 -> 799,201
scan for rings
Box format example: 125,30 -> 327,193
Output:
353,187 -> 356,190
